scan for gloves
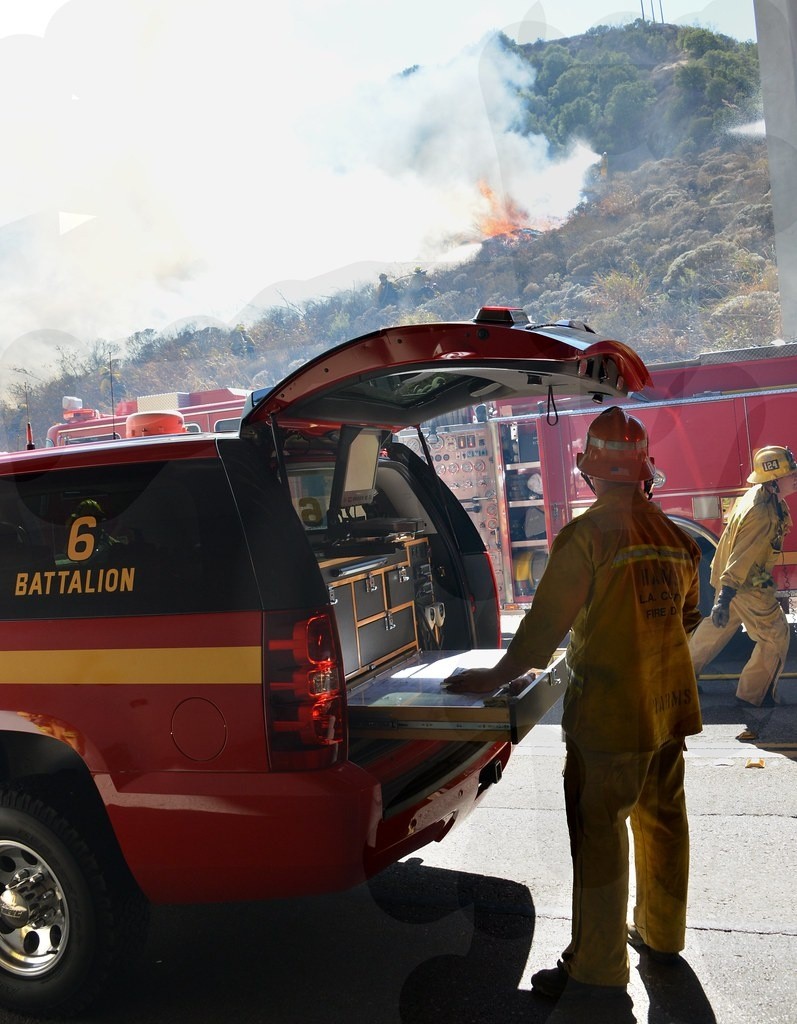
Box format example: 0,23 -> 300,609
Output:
711,586 -> 737,628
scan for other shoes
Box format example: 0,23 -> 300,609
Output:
531,959 -> 627,999
625,922 -> 646,946
738,696 -> 776,707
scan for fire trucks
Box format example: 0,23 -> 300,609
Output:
0,302 -> 650,1013
45,337 -> 797,656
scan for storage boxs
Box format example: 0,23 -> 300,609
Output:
313,538 -> 442,685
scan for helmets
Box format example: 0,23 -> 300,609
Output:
747,446 -> 797,483
575,406 -> 657,483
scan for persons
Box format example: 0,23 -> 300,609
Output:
441,406 -> 702,994
686,444 -> 797,708
